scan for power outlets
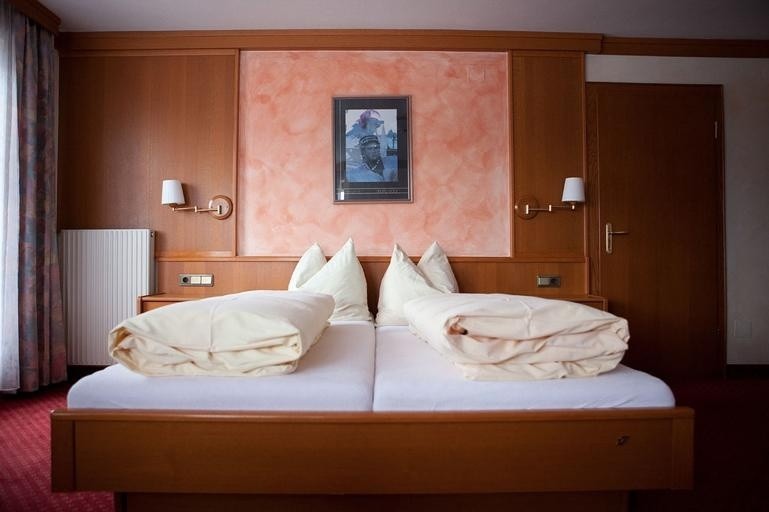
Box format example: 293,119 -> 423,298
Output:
536,274 -> 562,287
179,274 -> 215,287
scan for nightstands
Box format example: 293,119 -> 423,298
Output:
134,292 -> 196,313
548,295 -> 609,313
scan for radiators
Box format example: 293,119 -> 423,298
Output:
59,228 -> 152,366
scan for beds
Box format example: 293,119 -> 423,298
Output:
52,319 -> 698,502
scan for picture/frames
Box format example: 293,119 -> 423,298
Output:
332,96 -> 412,204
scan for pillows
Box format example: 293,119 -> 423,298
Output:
286,239 -> 372,320
375,242 -> 454,324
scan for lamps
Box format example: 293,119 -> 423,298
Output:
163,180 -> 232,219
517,179 -> 583,217
